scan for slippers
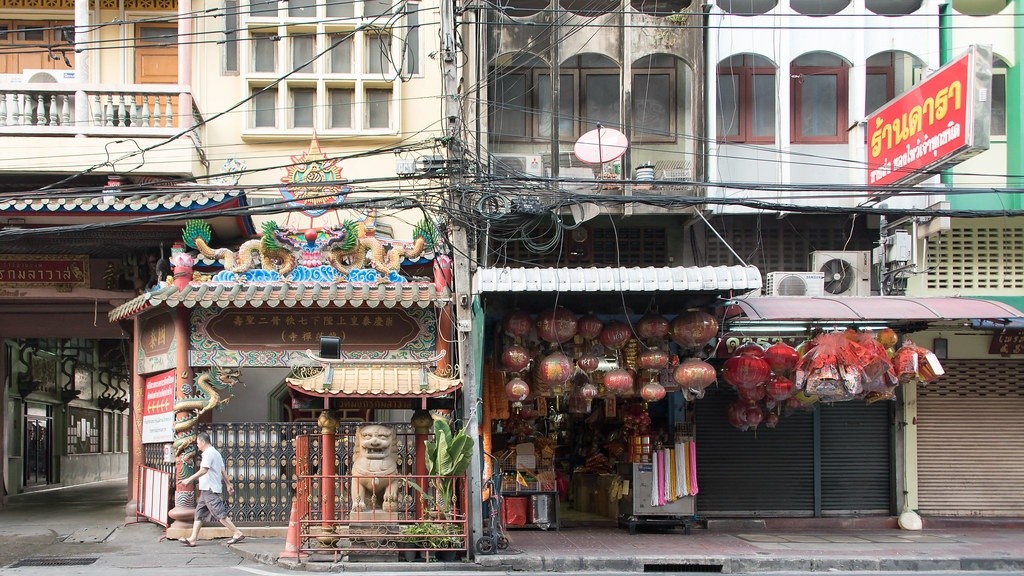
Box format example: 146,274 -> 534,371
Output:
227,535 -> 245,543
179,537 -> 195,547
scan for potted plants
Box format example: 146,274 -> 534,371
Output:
399,420 -> 474,562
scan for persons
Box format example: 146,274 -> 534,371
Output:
176,432 -> 245,547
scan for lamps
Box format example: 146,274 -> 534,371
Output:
320,335 -> 341,358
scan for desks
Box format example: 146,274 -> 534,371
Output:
502,491 -> 560,530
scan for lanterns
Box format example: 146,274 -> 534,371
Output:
502,307 -> 945,440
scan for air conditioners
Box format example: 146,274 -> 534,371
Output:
490,154 -> 542,187
806,249 -> 872,299
23,69 -> 76,107
766,271 -> 825,296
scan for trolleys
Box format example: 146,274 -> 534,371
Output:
476,447 -> 516,554
618,458 -> 694,536
